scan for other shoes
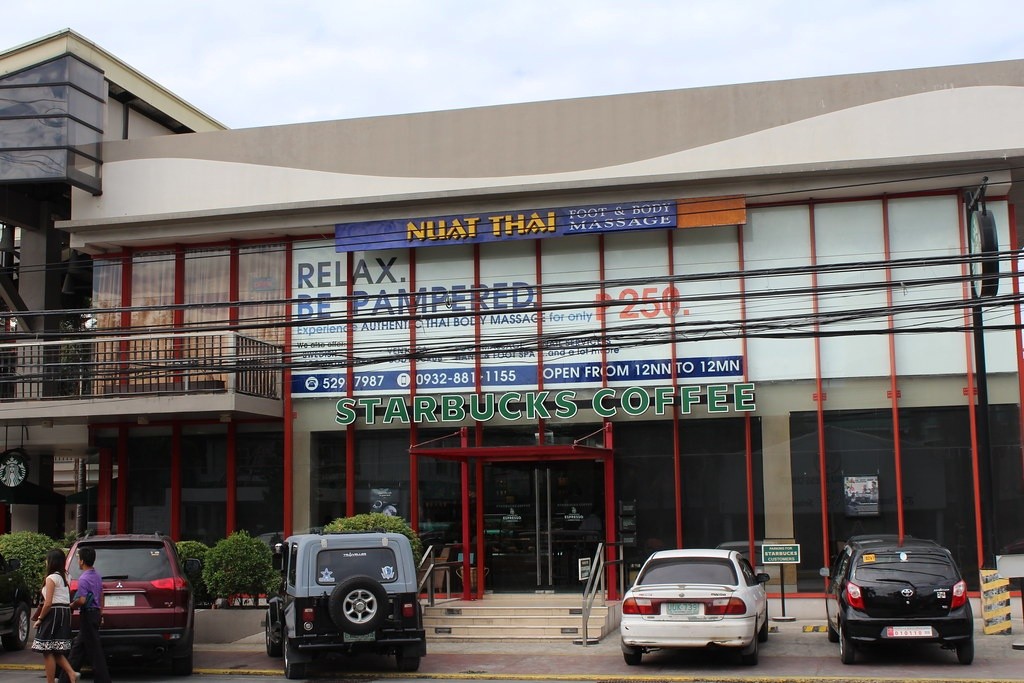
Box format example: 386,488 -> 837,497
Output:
75,672 -> 81,683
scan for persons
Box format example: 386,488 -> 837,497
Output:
455,489 -> 483,545
29,549 -> 81,683
57,547 -> 113,683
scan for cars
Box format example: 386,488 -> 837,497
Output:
0,554 -> 36,651
620,549 -> 771,668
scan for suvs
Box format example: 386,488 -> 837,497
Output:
820,535 -> 977,664
264,534 -> 426,679
65,531 -> 201,675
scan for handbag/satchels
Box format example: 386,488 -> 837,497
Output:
73,591 -> 94,608
31,604 -> 43,621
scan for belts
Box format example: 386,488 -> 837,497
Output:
81,608 -> 100,611
